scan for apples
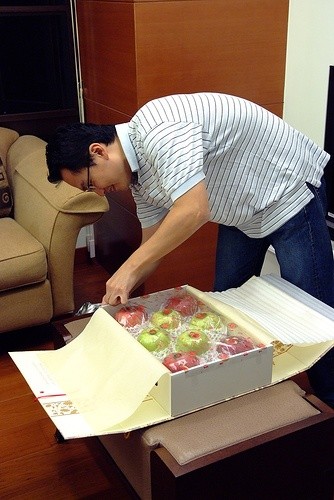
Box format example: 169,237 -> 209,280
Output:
116,292 -> 255,373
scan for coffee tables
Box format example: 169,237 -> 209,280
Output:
55,320 -> 333,500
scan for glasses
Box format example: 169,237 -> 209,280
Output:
86,167 -> 97,193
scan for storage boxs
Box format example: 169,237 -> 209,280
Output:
7,273 -> 333,440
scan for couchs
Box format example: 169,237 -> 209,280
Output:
1,127 -> 114,339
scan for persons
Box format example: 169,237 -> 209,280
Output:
44,91 -> 334,414
